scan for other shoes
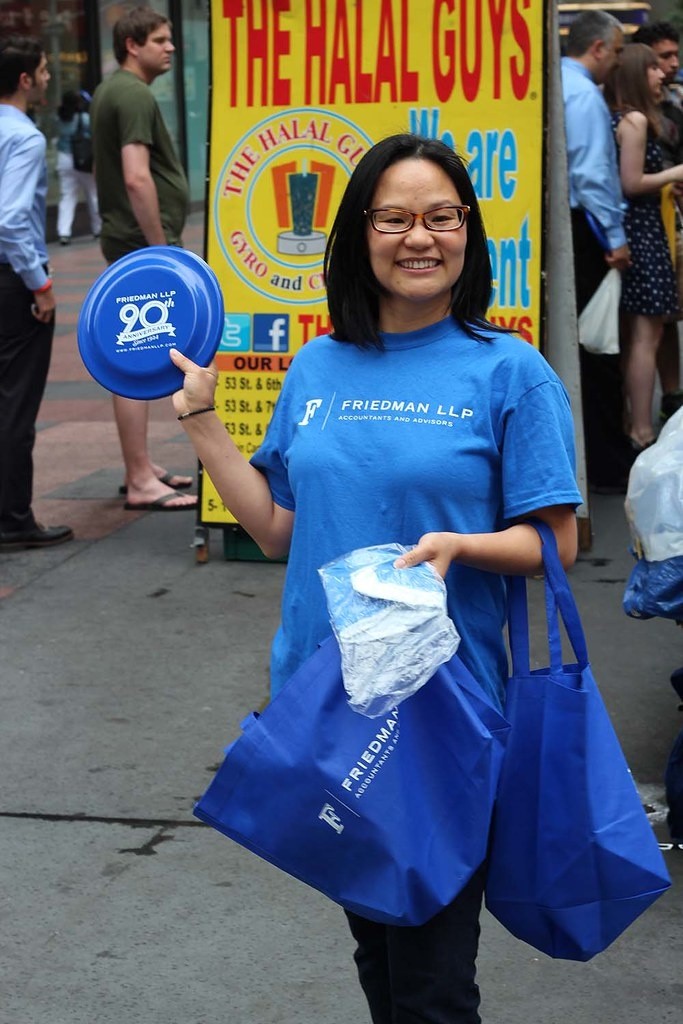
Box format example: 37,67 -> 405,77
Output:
659,391 -> 682,423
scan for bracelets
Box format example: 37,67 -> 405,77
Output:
177,406 -> 215,421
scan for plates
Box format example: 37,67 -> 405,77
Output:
77,247 -> 225,401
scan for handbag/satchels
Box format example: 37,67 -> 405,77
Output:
484,517 -> 673,962
70,112 -> 93,173
192,609 -> 513,933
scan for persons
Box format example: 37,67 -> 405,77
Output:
560,11 -> 683,452
0,38 -> 75,554
90,6 -> 200,512
169,135 -> 584,1024
56,91 -> 102,244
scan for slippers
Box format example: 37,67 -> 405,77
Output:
119,470 -> 194,496
123,490 -> 199,511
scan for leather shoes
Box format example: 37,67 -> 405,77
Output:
0,526 -> 75,551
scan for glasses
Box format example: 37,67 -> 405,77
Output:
363,205 -> 471,234
